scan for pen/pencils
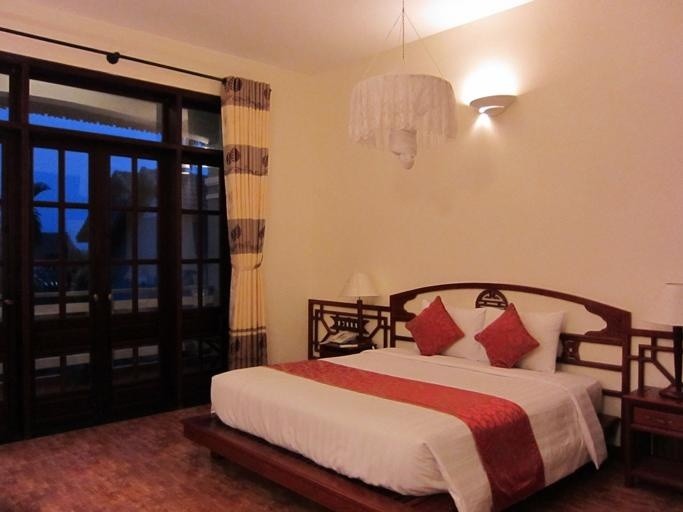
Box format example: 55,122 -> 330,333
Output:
336,345 -> 359,348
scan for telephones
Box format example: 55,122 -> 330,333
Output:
329,331 -> 357,343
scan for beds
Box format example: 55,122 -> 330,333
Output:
177,282 -> 632,510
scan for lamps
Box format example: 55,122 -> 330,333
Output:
344,1 -> 458,172
470,95 -> 516,119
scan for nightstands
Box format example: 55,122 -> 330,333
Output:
621,384 -> 683,495
319,335 -> 371,359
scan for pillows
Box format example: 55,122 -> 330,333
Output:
401,290 -> 567,378
